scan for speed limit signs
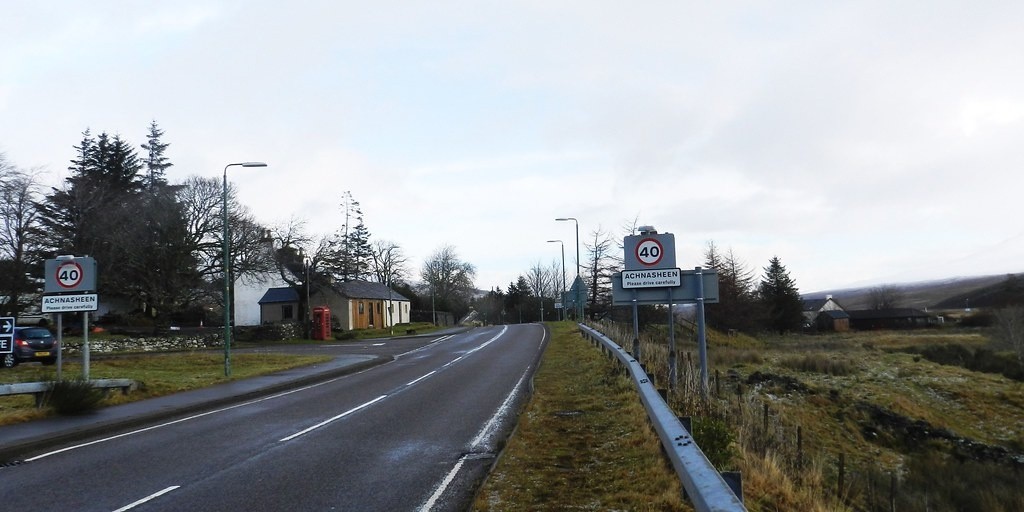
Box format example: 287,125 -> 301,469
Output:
43,257 -> 96,293
637,238 -> 663,265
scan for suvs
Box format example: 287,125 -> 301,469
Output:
0,325 -> 58,369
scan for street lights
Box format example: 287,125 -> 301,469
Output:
223,162 -> 267,380
546,239 -> 567,322
555,217 -> 580,323
430,261 -> 438,328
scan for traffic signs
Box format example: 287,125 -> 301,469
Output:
0,318 -> 15,336
